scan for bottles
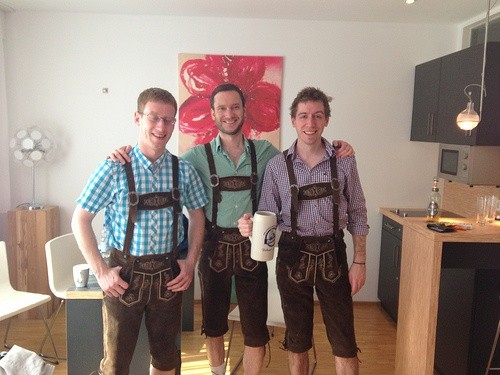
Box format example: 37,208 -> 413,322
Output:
425,177 -> 442,223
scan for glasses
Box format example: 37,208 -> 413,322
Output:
141,111 -> 176,126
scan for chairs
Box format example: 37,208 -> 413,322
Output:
38,233 -> 89,360
223,246 -> 317,375
0,240 -> 60,366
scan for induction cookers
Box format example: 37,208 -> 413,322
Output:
390,208 -> 463,217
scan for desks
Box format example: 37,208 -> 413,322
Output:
65,266 -> 194,375
393,216 -> 500,375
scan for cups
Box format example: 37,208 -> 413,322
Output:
248,211 -> 277,262
73,264 -> 89,288
475,194 -> 497,225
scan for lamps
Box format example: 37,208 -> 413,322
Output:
9,127 -> 55,210
457,0 -> 492,131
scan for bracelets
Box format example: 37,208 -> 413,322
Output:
353,261 -> 365,265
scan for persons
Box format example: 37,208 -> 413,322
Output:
238,88 -> 370,375
71,87 -> 208,375
107,83 -> 355,375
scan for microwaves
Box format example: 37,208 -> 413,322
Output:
437,143 -> 500,188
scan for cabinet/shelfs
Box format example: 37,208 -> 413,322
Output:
7,206 -> 60,320
409,41 -> 500,147
377,214 -> 403,328
